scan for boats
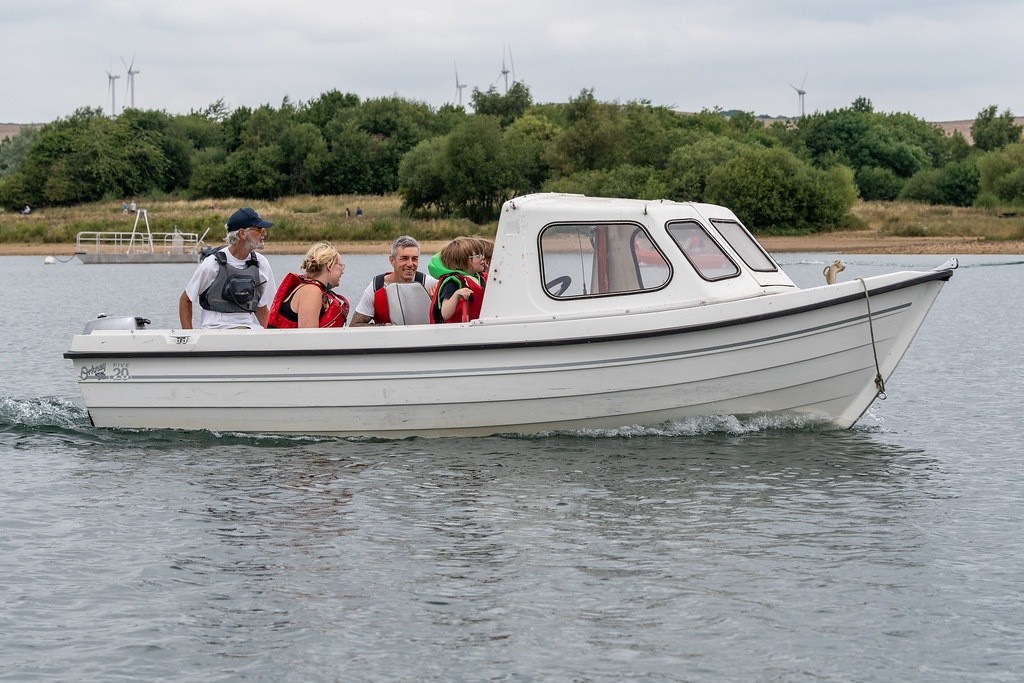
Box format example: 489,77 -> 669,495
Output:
72,208 -> 214,264
61,190 -> 959,431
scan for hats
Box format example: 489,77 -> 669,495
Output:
226,207 -> 274,232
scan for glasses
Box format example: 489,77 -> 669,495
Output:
239,227 -> 267,236
335,263 -> 346,269
469,254 -> 485,260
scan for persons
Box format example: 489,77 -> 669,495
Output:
21,205 -> 31,214
264,241 -> 349,328
345,206 -> 362,220
122,200 -> 136,214
349,235 -> 494,327
179,208 -> 270,329
